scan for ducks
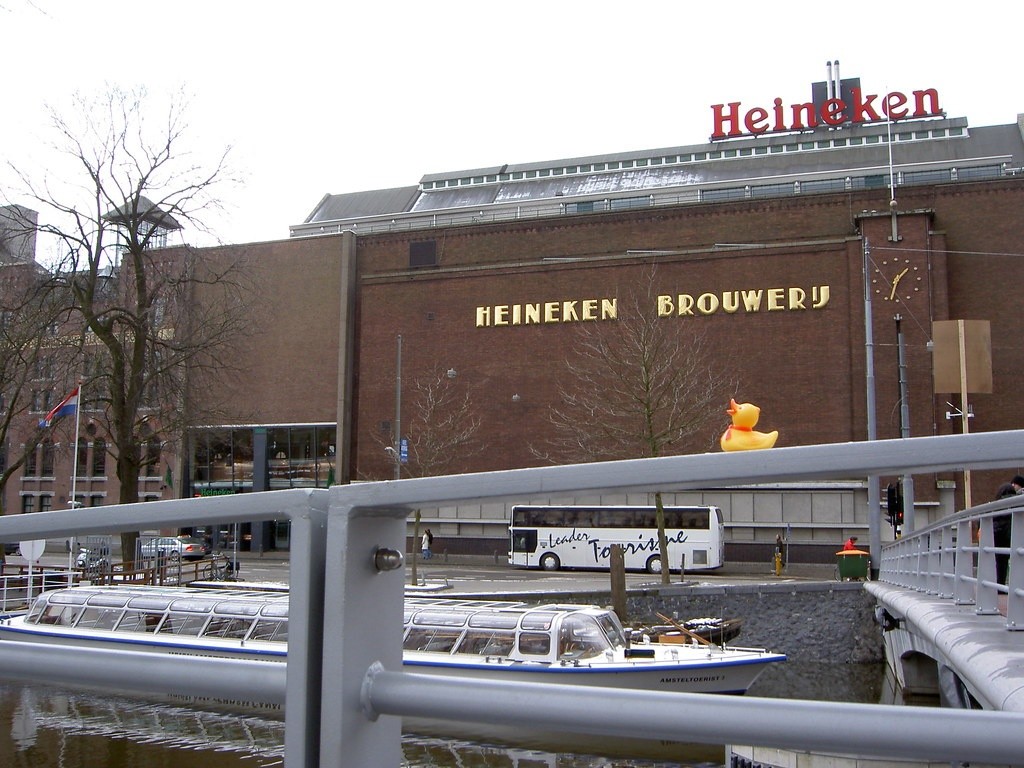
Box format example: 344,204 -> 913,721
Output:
719,398 -> 778,454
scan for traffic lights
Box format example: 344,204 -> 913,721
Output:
897,497 -> 904,524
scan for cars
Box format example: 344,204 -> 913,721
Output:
140,535 -> 207,561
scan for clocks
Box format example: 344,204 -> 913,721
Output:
872,256 -> 923,304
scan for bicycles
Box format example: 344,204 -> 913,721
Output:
203,553 -> 240,581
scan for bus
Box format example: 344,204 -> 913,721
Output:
507,505 -> 725,575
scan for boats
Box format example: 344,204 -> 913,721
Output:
1,380 -> 788,760
724,742 -> 981,768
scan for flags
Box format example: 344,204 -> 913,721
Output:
39,387 -> 79,429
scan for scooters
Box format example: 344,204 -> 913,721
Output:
77,543 -> 108,573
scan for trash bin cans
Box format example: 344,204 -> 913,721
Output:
836,549 -> 870,582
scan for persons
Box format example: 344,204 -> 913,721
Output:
421,529 -> 433,559
842,536 -> 859,553
978,476 -> 1024,593
776,534 -> 786,566
